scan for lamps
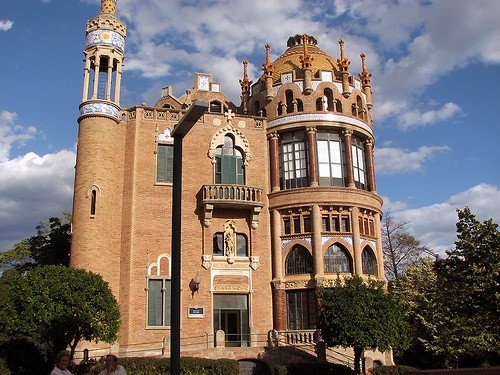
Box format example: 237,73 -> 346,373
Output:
193,271 -> 201,293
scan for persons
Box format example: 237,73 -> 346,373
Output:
99,355 -> 128,375
50,350 -> 72,375
